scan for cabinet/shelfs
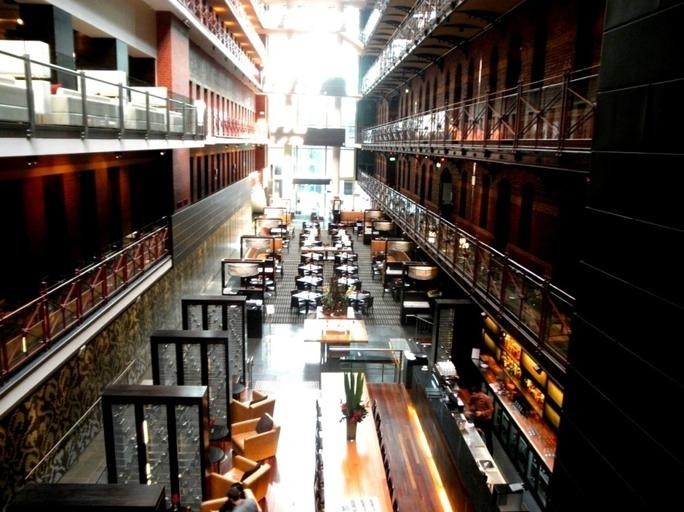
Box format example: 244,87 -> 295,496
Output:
480,310 -> 564,437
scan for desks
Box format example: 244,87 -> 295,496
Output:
301,317 -> 369,372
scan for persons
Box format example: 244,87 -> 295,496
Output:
464,386 -> 495,457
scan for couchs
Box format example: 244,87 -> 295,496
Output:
0,74 -> 185,135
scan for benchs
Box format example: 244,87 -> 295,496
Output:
325,346 -> 403,386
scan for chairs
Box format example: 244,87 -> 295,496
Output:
195,372 -> 281,512
313,370 -> 400,512
218,202 -> 445,335
